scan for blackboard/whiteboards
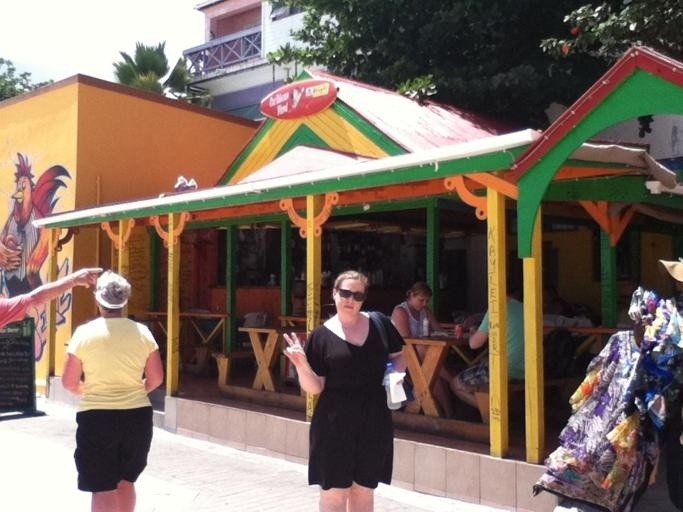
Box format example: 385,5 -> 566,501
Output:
0,317 -> 36,414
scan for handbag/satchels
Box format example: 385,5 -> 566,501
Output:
374,313 -> 414,412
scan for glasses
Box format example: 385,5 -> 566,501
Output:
336,286 -> 365,303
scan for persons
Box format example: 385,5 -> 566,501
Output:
60,269 -> 166,512
280,270 -> 410,511
0,267 -> 104,334
389,281 -> 456,418
448,276 -> 524,427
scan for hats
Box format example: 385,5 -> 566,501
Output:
93,269 -> 132,311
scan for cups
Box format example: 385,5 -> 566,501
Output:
455,323 -> 465,340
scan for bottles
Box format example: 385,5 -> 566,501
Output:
383,361 -> 403,410
422,315 -> 431,337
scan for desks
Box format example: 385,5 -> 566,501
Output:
146,307 -> 634,431
